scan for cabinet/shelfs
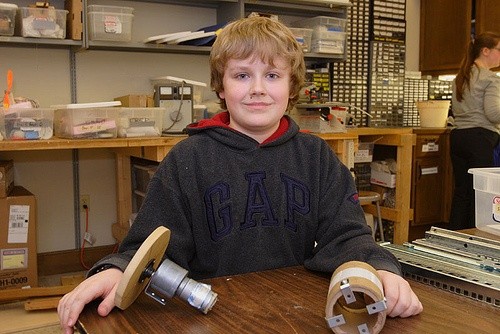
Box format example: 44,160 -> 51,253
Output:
0,0 -> 347,63
418,0 -> 500,76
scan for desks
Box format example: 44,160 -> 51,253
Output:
74,264 -> 500,334
372,127 -> 456,244
0,125 -> 417,252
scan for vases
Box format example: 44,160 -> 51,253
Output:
416,99 -> 452,128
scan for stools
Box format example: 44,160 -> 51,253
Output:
357,192 -> 385,243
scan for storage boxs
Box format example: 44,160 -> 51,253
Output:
0,160 -> 38,305
468,167 -> 500,237
0,3 -> 135,41
0,75 -> 207,141
353,142 -> 396,231
286,15 -> 348,55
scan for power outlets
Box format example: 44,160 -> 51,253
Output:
79,194 -> 90,212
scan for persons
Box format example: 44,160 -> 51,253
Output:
57,15 -> 423,334
446,33 -> 500,229
